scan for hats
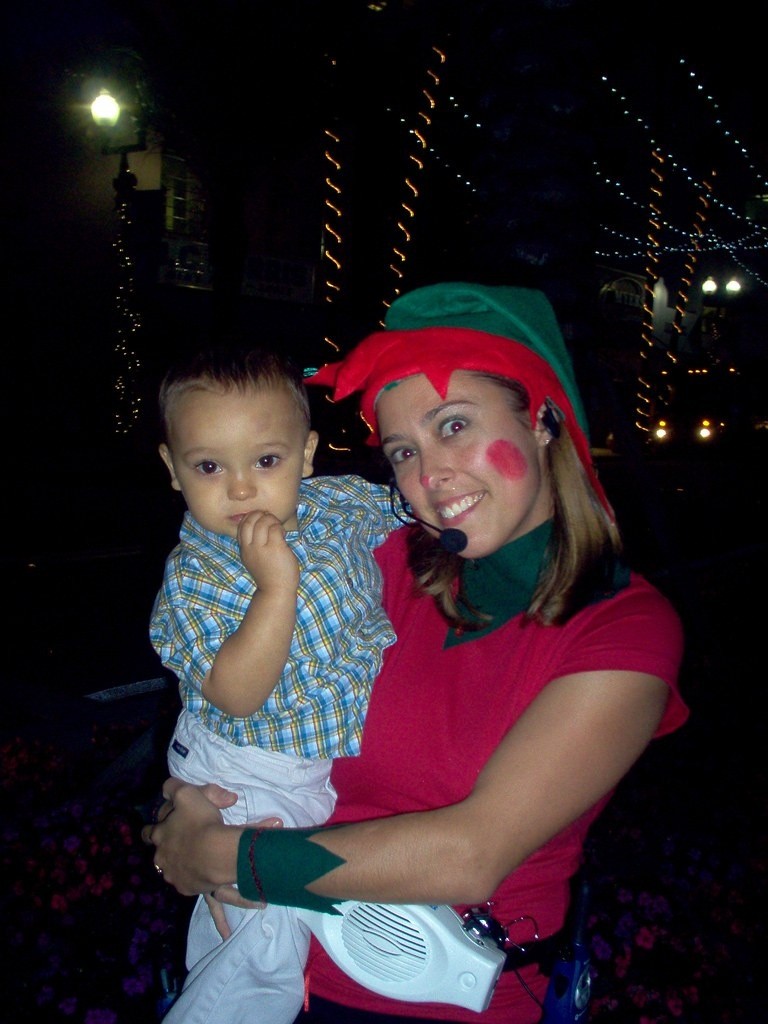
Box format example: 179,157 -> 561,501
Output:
302,282 -> 616,527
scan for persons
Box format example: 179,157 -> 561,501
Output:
150,336 -> 419,1024
141,283 -> 691,1024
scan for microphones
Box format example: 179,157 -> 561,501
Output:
396,492 -> 469,554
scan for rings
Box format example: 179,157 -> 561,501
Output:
154,863 -> 163,873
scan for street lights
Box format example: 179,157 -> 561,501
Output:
83,76 -> 146,660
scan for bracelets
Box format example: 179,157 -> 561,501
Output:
249,828 -> 267,908
236,822 -> 352,915
